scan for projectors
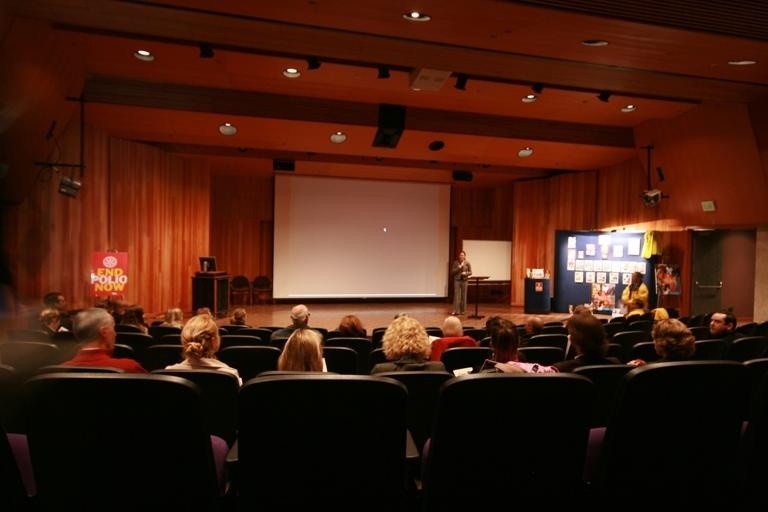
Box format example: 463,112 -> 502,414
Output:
408,69 -> 453,94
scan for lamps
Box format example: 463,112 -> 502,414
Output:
304,56 -> 320,70
31,161 -> 87,200
597,91 -> 612,101
530,82 -> 544,94
199,41 -> 214,57
452,73 -> 472,91
643,144 -> 663,207
378,65 -> 390,78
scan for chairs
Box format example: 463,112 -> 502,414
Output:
230,275 -> 251,305
252,276 -> 273,305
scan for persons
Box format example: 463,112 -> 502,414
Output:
277,327 -> 322,371
164,314 -> 243,386
452,251 -> 471,315
63,305 -> 147,373
621,272 -> 650,310
272,305 -> 321,338
40,292 -> 251,334
340,303 -> 737,375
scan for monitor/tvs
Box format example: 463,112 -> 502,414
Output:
199,256 -> 216,272
